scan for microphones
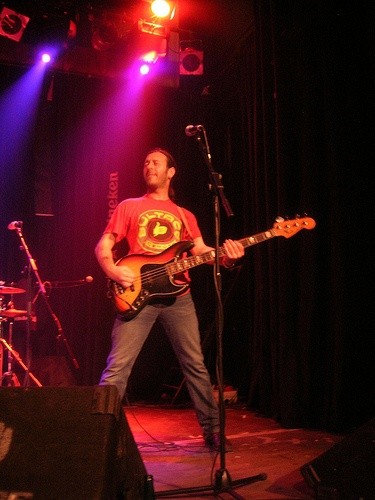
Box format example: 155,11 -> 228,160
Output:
7,221 -> 23,230
185,124 -> 203,137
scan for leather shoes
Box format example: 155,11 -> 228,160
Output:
205,433 -> 234,452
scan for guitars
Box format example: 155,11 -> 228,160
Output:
110,211 -> 317,321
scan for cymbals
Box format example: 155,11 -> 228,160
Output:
0,309 -> 28,318
0,286 -> 28,296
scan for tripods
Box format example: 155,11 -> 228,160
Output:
156,128 -> 266,500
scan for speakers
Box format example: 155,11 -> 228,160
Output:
0,383 -> 155,500
299,422 -> 375,500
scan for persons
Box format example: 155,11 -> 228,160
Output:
95,149 -> 244,452
